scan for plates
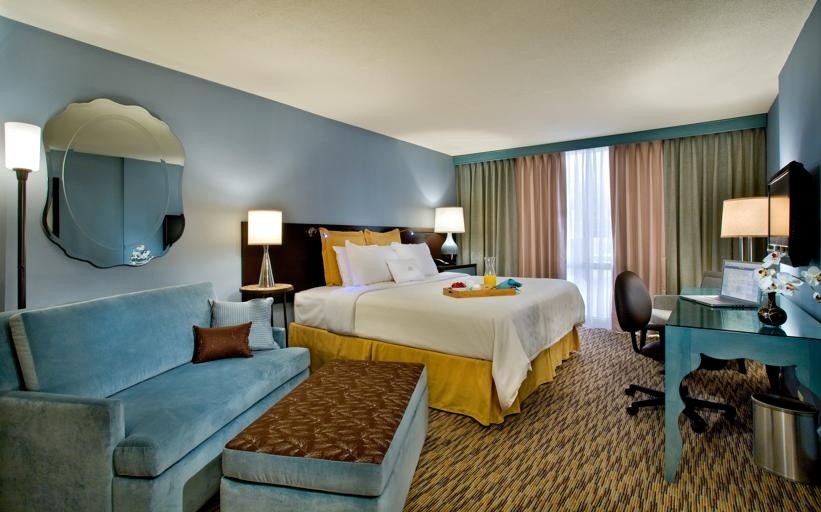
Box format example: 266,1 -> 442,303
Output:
452,286 -> 471,292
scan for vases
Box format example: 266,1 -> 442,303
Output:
758,292 -> 787,327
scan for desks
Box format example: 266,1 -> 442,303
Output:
663,285 -> 821,484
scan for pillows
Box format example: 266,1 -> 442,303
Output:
208,297 -> 281,352
192,321 -> 253,364
319,227 -> 439,287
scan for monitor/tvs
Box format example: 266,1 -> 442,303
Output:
163,214 -> 185,250
767,159 -> 821,267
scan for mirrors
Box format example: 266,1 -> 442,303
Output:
40,97 -> 186,269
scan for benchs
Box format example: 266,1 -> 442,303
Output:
220,360 -> 429,512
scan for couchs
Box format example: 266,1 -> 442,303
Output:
0,282 -> 310,512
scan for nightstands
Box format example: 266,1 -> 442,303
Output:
436,263 -> 477,276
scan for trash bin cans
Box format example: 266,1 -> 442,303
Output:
751,392 -> 819,483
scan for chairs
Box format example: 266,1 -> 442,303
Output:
650,271 -> 723,326
614,271 -> 736,434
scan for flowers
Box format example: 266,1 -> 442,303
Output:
754,251 -> 821,304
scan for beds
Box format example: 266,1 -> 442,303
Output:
240,221 -> 585,426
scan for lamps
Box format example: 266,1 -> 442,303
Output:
247,209 -> 283,288
720,196 -> 769,262
769,195 -> 789,237
5,121 -> 42,309
434,207 -> 465,264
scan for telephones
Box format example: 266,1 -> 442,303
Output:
435,258 -> 450,266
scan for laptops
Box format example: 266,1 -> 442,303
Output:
679,259 -> 766,307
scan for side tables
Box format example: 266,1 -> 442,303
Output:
239,283 -> 294,348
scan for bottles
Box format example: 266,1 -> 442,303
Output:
483,256 -> 497,290
